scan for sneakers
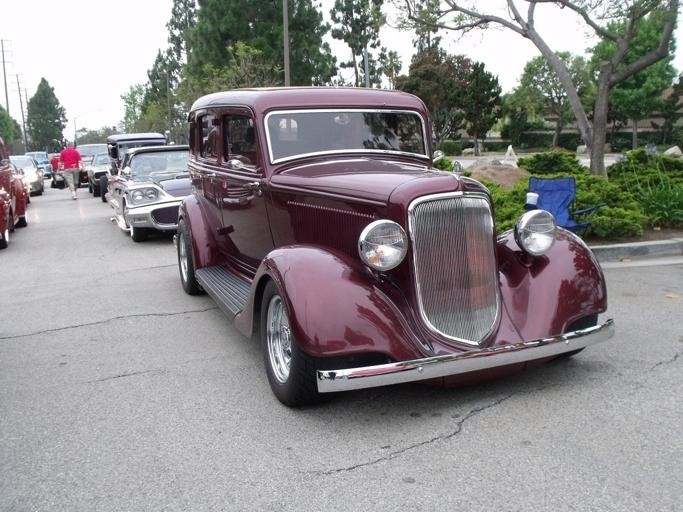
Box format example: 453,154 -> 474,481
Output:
73,193 -> 79,200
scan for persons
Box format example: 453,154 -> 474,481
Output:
58,142 -> 84,200
50,153 -> 59,172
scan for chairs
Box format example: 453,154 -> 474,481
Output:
523,177 -> 606,240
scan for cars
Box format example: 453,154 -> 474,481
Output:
52,133 -> 172,200
104,145 -> 199,239
0,137 -> 55,245
170,86 -> 616,409
